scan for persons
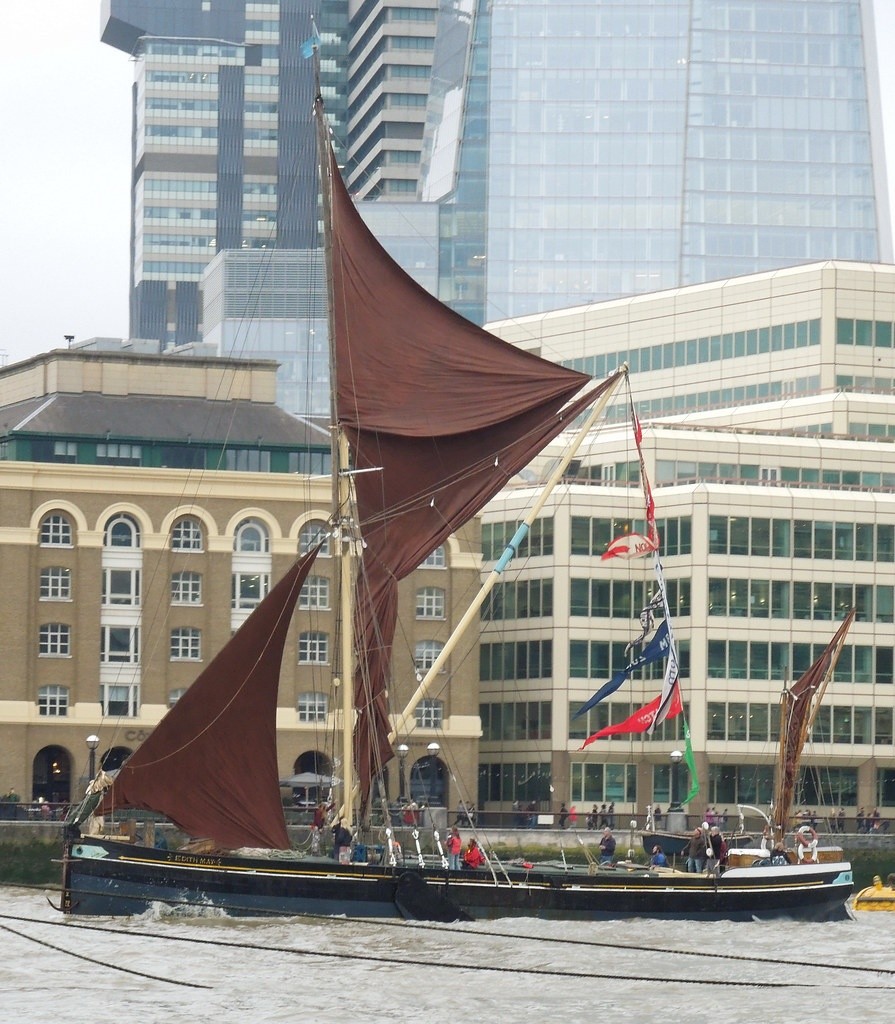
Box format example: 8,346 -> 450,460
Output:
281,793 -> 428,826
452,800 -> 476,828
649,845 -> 670,874
770,842 -> 792,866
599,827 -> 616,864
837,807 -> 847,833
444,826 -> 462,870
586,801 -> 616,831
512,800 -> 538,829
461,839 -> 486,870
792,810 -> 818,833
392,835 -> 401,860
331,816 -> 353,862
887,873 -> 895,891
704,807 -> 728,831
681,826 -> 727,874
654,804 -> 662,832
828,808 -> 838,834
559,802 -> 577,829
0,788 -> 70,821
855,807 -> 889,834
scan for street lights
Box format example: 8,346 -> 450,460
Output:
425,743 -> 442,807
85,734 -> 101,781
666,750 -> 685,812
396,743 -> 410,807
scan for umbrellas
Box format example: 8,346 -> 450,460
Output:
275,771 -> 338,812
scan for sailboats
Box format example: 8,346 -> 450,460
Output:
43,47 -> 861,925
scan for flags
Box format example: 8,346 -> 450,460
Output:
299,19 -> 322,59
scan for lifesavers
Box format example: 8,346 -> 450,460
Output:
797,825 -> 819,848
797,843 -> 818,864
